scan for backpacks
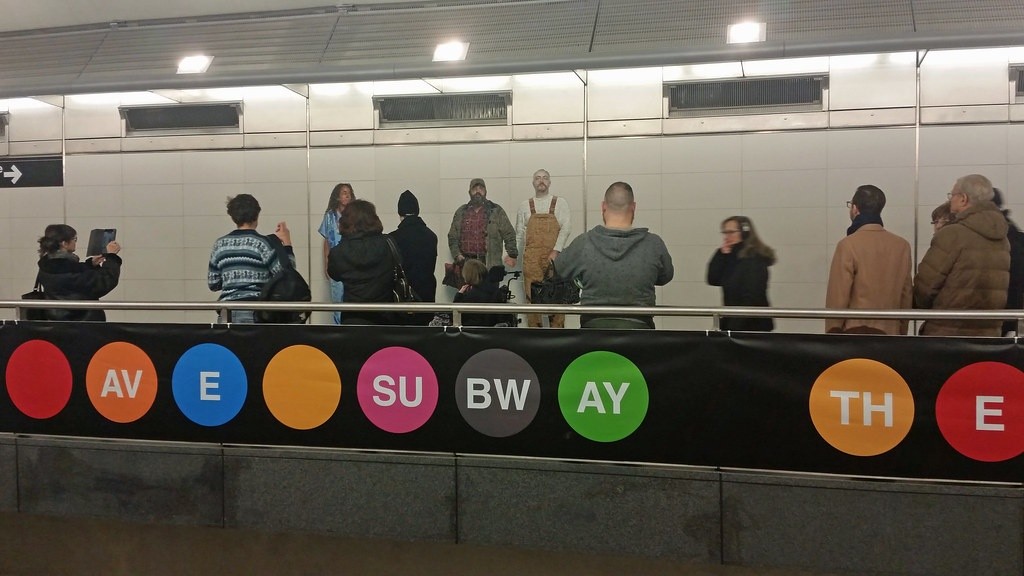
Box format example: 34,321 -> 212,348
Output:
253,234 -> 312,324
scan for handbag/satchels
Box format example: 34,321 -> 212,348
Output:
383,234 -> 424,321
530,258 -> 581,304
19,291 -> 49,320
442,257 -> 465,291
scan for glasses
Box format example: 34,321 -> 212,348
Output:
847,200 -> 854,208
721,229 -> 742,235
69,236 -> 78,242
932,219 -> 949,226
948,193 -> 961,201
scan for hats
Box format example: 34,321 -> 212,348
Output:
397,190 -> 420,215
469,178 -> 486,189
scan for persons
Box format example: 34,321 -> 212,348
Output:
389,190 -> 438,320
912,174 -> 1024,337
448,169 -> 573,328
318,183 -> 356,325
326,198 -> 400,325
825,185 -> 913,335
208,194 -> 296,324
36,224 -> 123,322
453,259 -> 490,327
705,215 -> 778,332
555,182 -> 674,329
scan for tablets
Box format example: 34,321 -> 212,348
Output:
101,229 -> 116,256
